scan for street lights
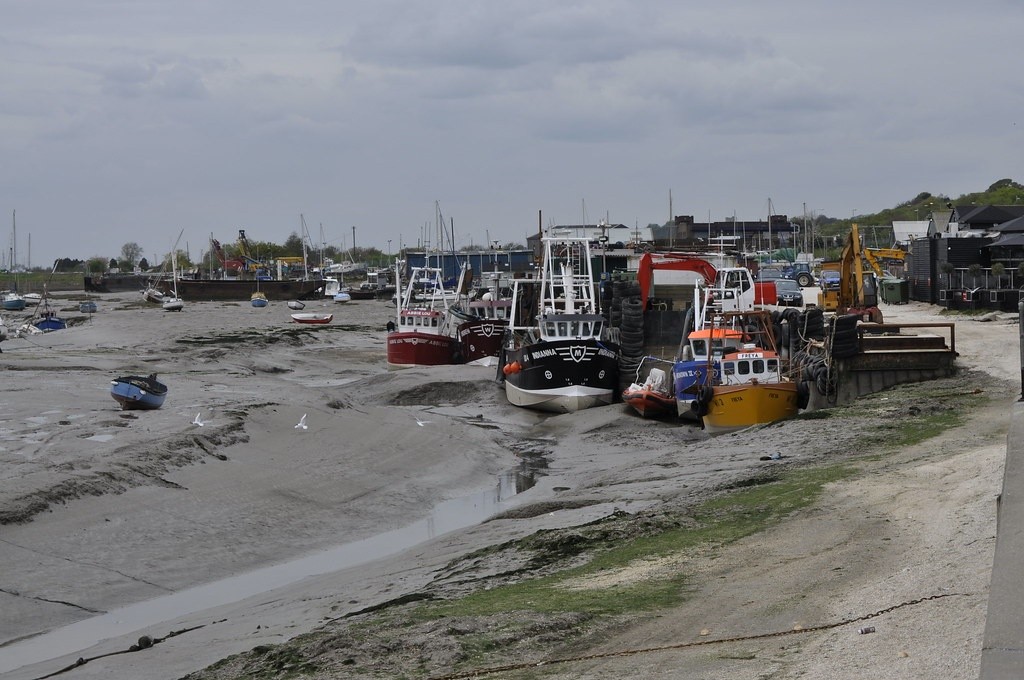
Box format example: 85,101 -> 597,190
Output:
811,208 -> 825,259
850,209 -> 856,218
388,239 -> 392,267
908,204 -> 942,222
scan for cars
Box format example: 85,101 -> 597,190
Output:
772,278 -> 803,307
755,268 -> 784,283
819,272 -> 840,290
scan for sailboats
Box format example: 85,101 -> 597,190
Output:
162,241 -> 183,313
15,258 -> 61,337
142,229 -> 186,303
19,234 -> 40,304
249,244 -> 269,307
0,248 -> 26,311
299,213 -> 617,415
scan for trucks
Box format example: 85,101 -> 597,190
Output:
756,263 -> 816,287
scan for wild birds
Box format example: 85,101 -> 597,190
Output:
409,413 -> 435,427
189,412 -> 205,428
293,412 -> 309,430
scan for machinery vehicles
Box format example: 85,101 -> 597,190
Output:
864,247 -> 904,285
804,222 -> 884,324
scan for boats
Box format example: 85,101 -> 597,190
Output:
621,354 -> 678,419
291,312 -> 333,323
32,284 -> 66,334
671,327 -> 743,417
79,301 -> 97,313
333,292 -> 350,303
109,374 -> 168,409
288,300 -> 305,310
697,343 -> 799,437
